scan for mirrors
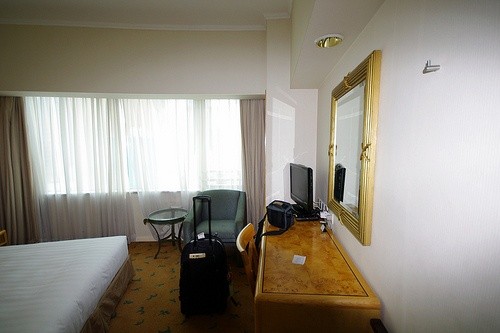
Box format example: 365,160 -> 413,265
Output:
327,50 -> 381,247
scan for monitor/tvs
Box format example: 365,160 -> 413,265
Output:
335,164 -> 346,203
289,162 -> 313,215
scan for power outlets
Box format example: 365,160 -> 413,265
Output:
318,199 -> 324,210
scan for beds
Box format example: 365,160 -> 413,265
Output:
0,236 -> 136,333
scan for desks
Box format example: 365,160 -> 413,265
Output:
147,208 -> 188,259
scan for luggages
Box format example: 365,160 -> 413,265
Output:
178,194 -> 231,318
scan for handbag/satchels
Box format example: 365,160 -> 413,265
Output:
254,200 -> 295,251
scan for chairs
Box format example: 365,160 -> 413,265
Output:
184,190 -> 246,270
236,222 -> 259,288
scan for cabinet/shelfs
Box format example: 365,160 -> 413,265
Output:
254,221 -> 381,333
